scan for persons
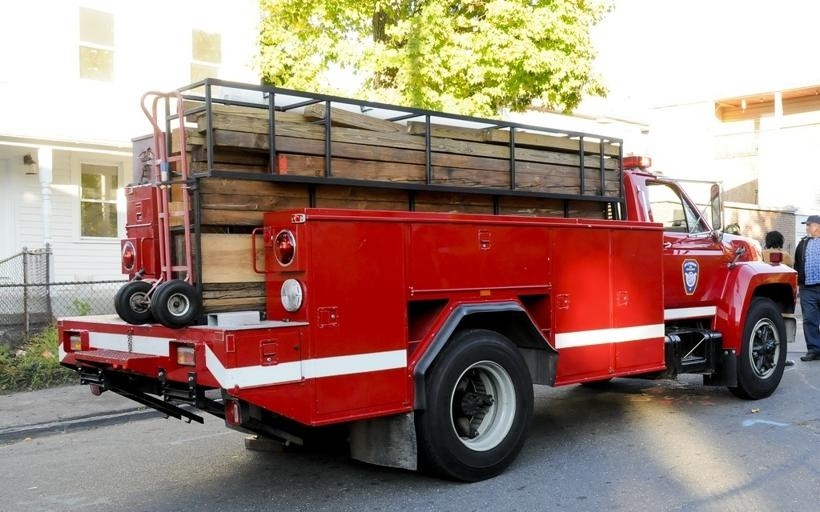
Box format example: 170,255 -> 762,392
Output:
761,230 -> 794,268
792,215 -> 820,361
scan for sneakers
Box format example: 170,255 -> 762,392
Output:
800,353 -> 819,361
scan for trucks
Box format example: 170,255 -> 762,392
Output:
51,73 -> 803,485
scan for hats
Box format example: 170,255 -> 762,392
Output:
801,215 -> 820,224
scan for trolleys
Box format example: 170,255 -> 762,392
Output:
113,86 -> 199,329
